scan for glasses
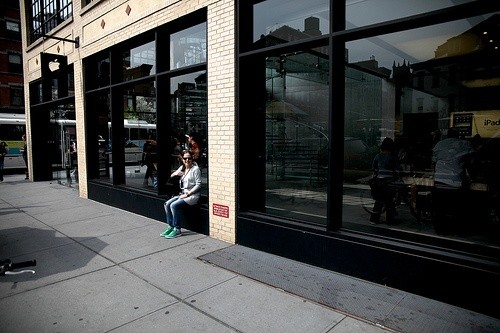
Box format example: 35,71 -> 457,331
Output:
183,157 -> 192,160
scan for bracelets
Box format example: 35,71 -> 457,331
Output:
186,192 -> 189,197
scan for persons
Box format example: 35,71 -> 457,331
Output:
19,134 -> 29,180
160,150 -> 202,238
141,133 -> 201,187
65,141 -> 78,185
0,137 -> 8,181
369,137 -> 404,225
430,126 -> 500,238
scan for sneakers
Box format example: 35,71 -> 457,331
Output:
159,225 -> 173,236
165,227 -> 183,238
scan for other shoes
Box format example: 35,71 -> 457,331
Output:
368,220 -> 375,224
